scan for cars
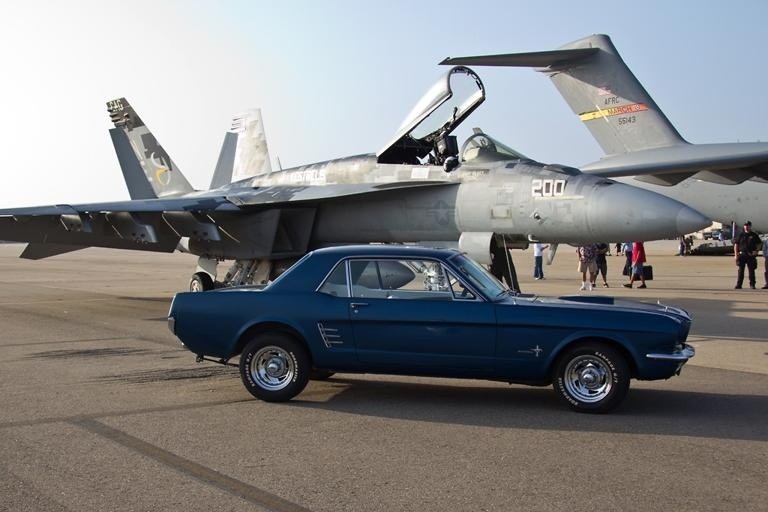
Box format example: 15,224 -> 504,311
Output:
168,245 -> 699,413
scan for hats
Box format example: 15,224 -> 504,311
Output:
743,220 -> 752,226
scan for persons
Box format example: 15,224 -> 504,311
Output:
534,243 -> 548,280
718,231 -> 723,241
680,234 -> 693,256
761,238 -> 768,289
733,220 -> 763,289
576,240 -> 646,291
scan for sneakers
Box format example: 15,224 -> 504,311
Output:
534,276 -> 546,281
761,285 -> 768,289
637,284 -> 646,289
623,284 -> 632,289
603,283 -> 608,288
735,285 -> 742,289
579,283 -> 597,292
750,285 -> 756,289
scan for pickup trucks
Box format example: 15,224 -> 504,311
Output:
703,227 -> 722,241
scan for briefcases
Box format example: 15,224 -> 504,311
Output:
629,265 -> 653,281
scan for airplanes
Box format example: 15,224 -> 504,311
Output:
439,31 -> 768,256
1,98 -> 715,294
